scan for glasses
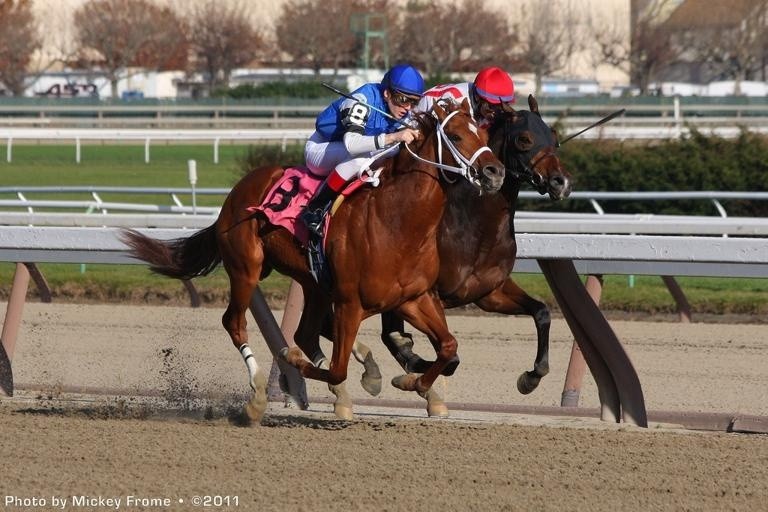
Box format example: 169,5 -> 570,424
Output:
387,68 -> 420,107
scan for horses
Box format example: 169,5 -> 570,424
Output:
321,94 -> 574,419
114,97 -> 508,429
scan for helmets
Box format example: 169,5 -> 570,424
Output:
381,67 -> 424,99
474,67 -> 515,104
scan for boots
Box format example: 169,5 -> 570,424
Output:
296,182 -> 338,237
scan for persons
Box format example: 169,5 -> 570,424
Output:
302,63 -> 424,238
394,65 -> 517,130
